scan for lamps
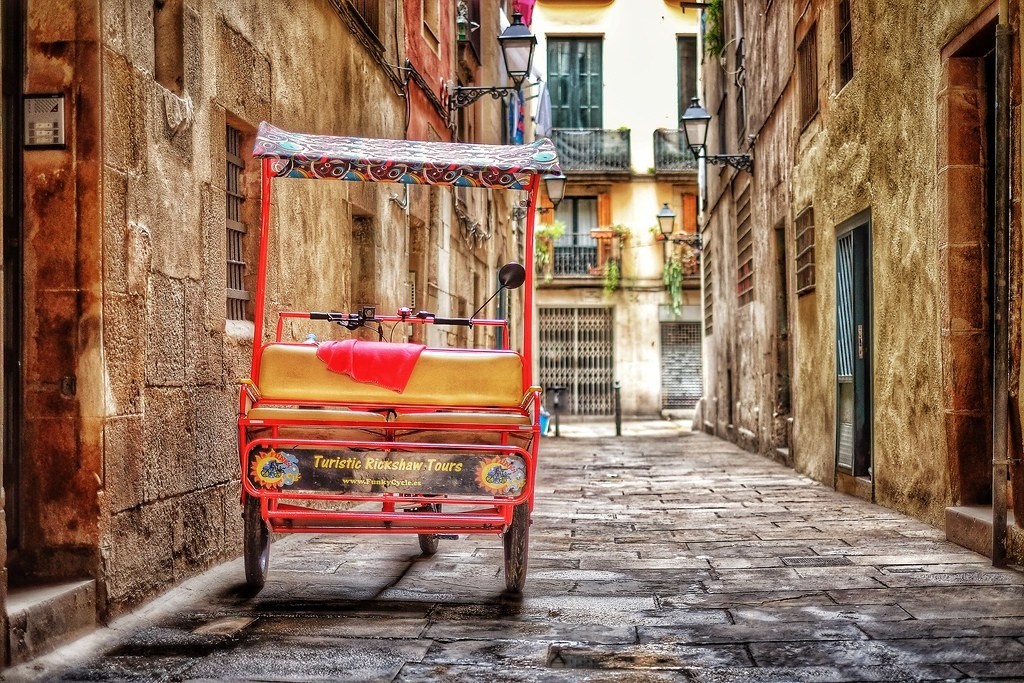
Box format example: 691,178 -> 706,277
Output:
679,94 -> 754,178
512,173 -> 567,221
656,202 -> 703,251
448,10 -> 538,111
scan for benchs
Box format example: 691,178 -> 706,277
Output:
241,342 -> 544,426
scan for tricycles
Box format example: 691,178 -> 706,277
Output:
234,119 -> 561,592
